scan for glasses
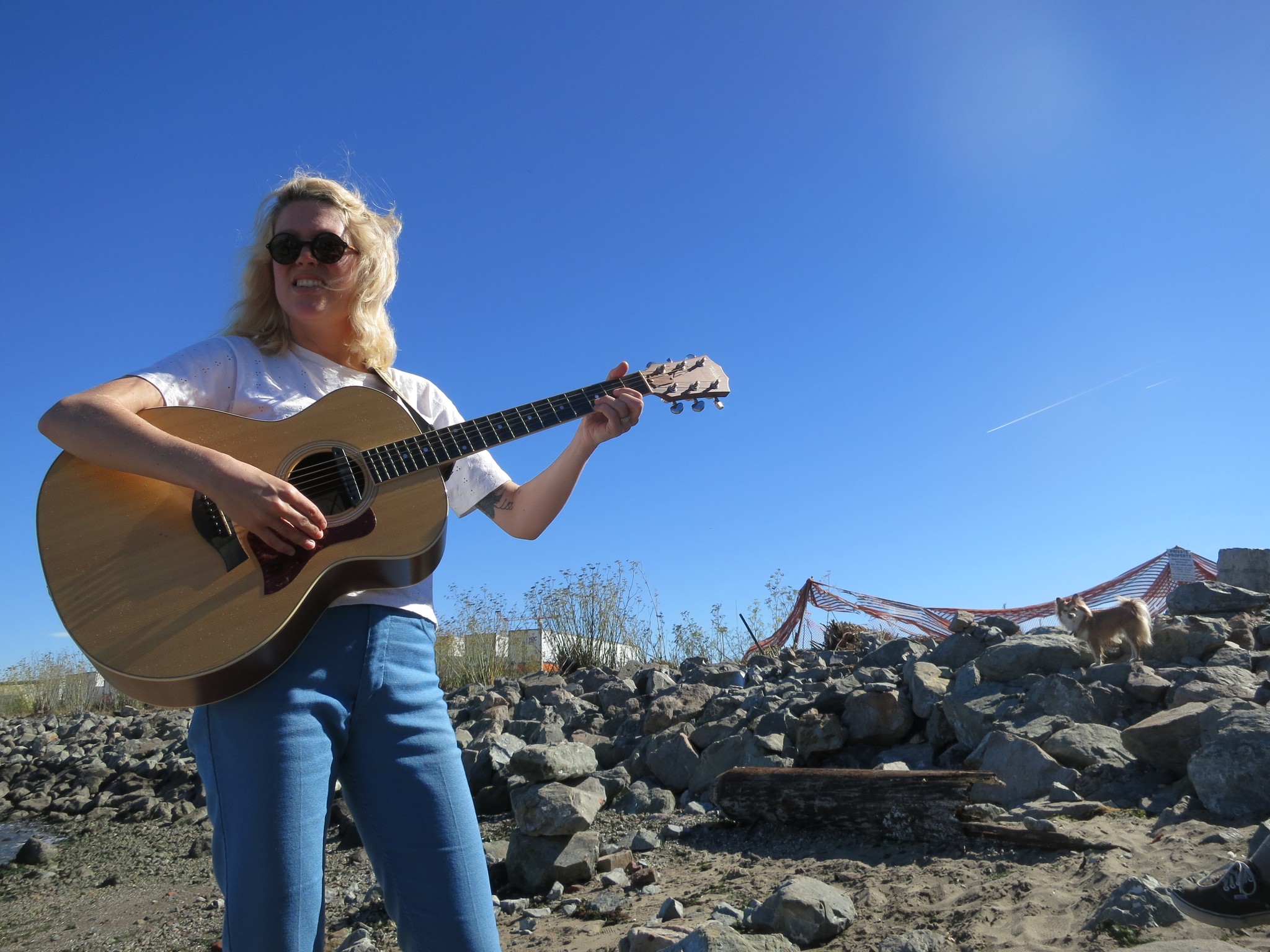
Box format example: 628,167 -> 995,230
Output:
266,231 -> 361,266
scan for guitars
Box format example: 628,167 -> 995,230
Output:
37,352 -> 730,707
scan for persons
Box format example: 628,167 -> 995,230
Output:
38,167 -> 645,952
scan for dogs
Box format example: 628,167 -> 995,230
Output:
1055,595 -> 1154,667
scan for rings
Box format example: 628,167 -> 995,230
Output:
620,416 -> 630,422
